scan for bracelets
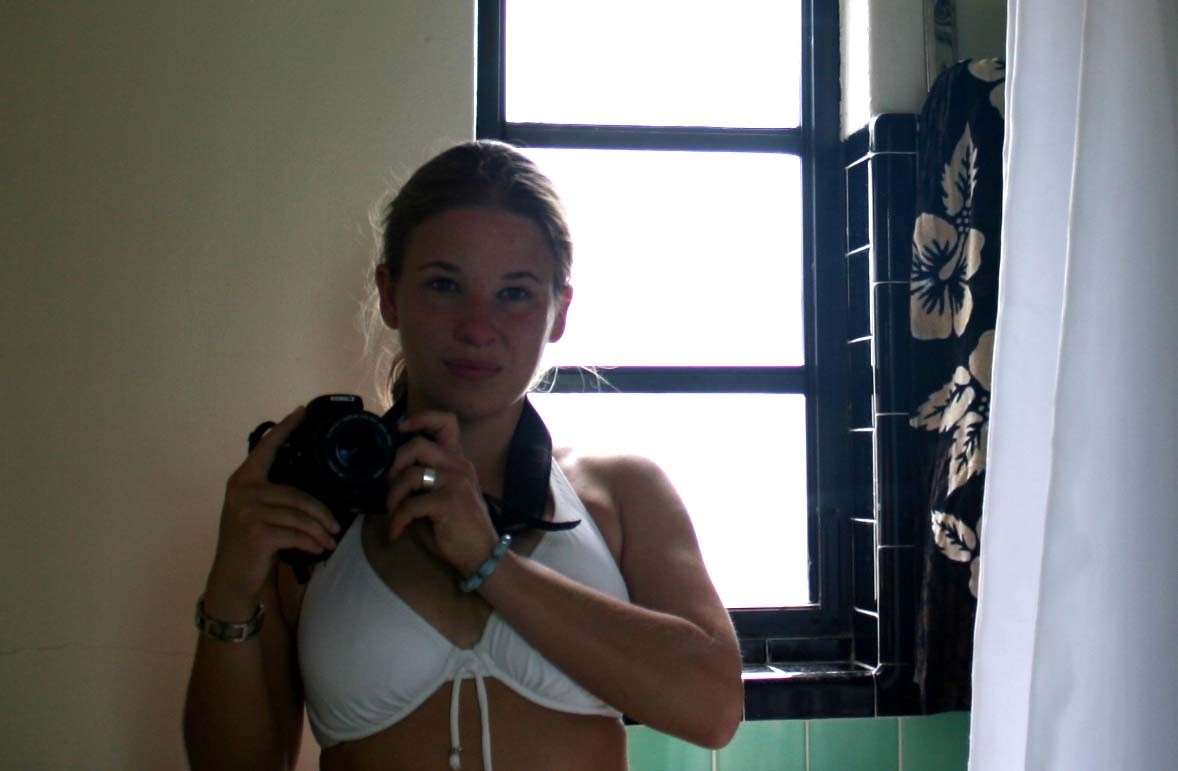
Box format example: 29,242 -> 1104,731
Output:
192,592 -> 264,645
460,534 -> 512,594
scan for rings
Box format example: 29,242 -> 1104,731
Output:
421,467 -> 435,489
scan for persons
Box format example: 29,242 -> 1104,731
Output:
182,140 -> 744,771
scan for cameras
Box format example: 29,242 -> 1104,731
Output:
264,394 -> 437,514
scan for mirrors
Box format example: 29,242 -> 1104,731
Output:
0,0 -> 1007,771
